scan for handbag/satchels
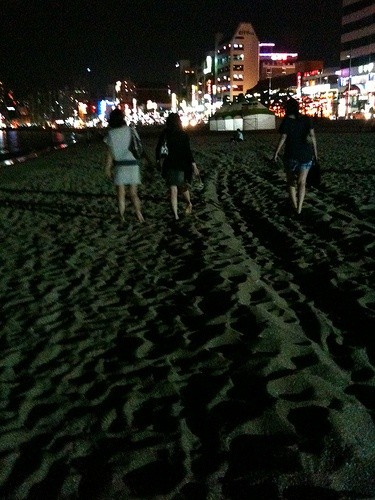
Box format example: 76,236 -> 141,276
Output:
128,125 -> 151,171
157,128 -> 168,169
308,161 -> 323,193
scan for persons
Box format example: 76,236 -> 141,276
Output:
154,112 -> 200,221
103,108 -> 146,224
272,98 -> 319,215
229,128 -> 244,147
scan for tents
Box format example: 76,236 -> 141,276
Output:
208,96 -> 276,131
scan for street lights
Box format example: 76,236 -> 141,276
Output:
346,53 -> 351,118
